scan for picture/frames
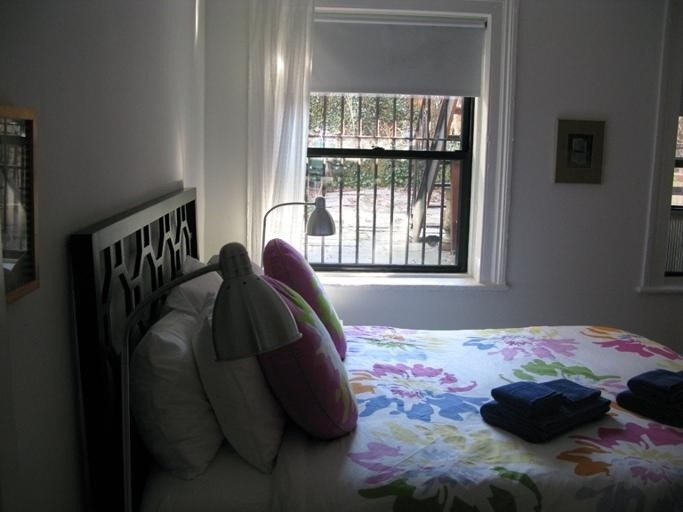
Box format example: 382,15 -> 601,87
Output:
0,104 -> 43,305
548,113 -> 611,188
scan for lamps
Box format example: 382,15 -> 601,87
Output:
260,195 -> 335,285
120,242 -> 305,512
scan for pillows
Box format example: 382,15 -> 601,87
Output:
198,306 -> 289,475
120,305 -> 224,484
266,237 -> 347,362
249,268 -> 359,439
164,251 -> 264,317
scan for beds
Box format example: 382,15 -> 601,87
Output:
65,190 -> 681,508
